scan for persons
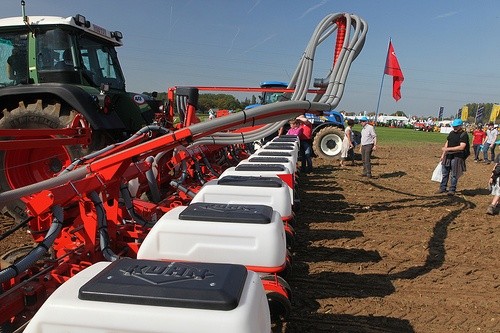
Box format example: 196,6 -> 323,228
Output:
486,121 -> 497,163
298,115 -> 312,170
487,152 -> 500,215
472,121 -> 484,161
361,116 -> 377,178
340,116 -> 356,168
285,117 -> 297,137
435,118 -> 472,195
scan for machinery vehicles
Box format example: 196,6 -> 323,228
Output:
0,1 -> 302,332
242,82 -> 357,164
415,122 -> 433,133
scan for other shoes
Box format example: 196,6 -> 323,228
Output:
359,173 -> 367,177
435,190 -> 447,194
486,206 -> 495,215
445,195 -> 453,203
474,158 -> 479,162
363,175 -> 371,179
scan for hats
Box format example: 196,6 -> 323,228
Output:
486,121 -> 494,125
360,117 -> 368,121
451,119 -> 463,126
477,122 -> 482,128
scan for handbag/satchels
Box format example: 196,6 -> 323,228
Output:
431,161 -> 443,183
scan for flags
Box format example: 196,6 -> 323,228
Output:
384,41 -> 404,102
